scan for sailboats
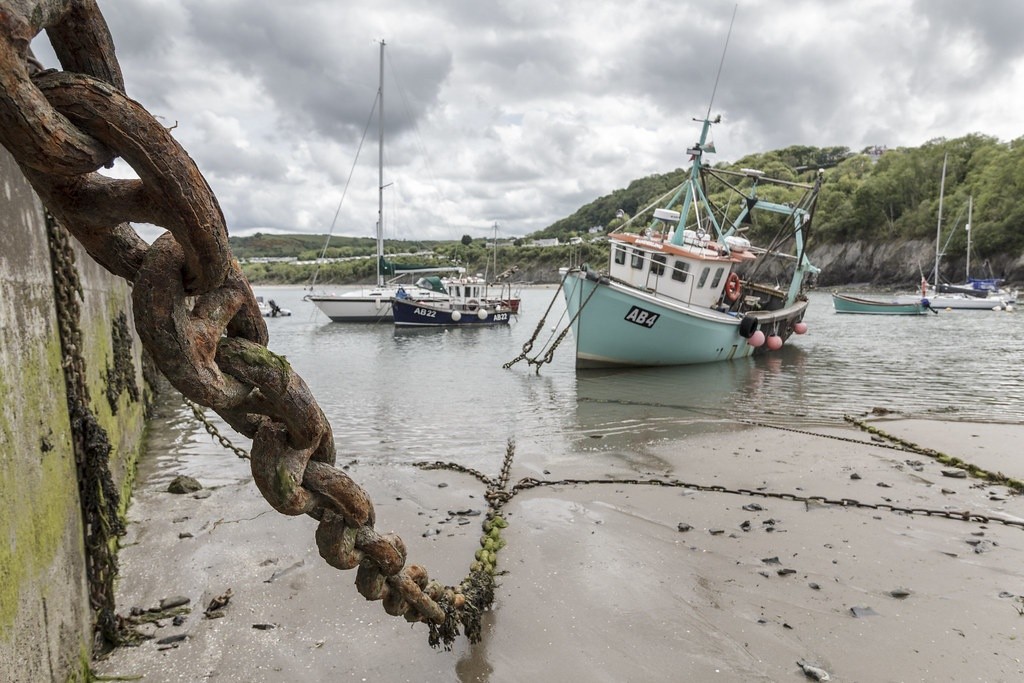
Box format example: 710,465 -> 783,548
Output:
300,38 -> 467,322
893,152 -> 1019,308
559,0 -> 828,374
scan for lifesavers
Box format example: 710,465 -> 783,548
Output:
725,272 -> 741,301
465,299 -> 480,312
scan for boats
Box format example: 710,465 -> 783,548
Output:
390,265 -> 522,332
832,290 -> 934,317
